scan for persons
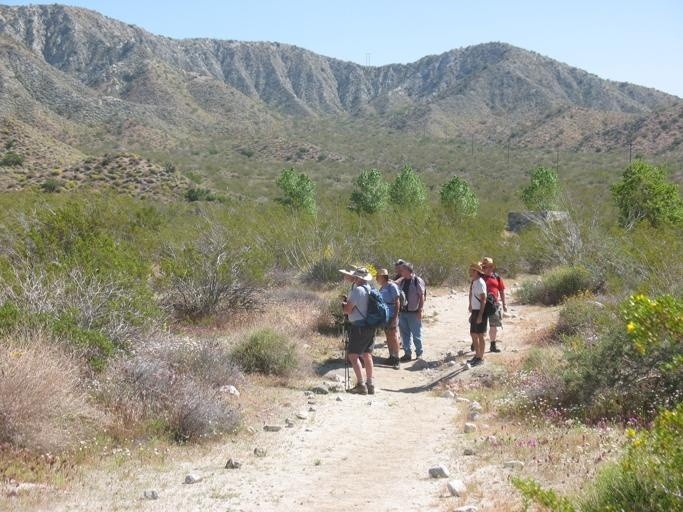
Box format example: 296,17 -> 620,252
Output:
344,267 -> 389,394
468,262 -> 488,366
375,269 -> 409,370
389,259 -> 413,345
338,265 -> 366,368
398,263 -> 425,361
469,256 -> 507,352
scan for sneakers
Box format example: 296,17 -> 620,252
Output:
471,343 -> 474,352
401,355 -> 411,361
385,356 -> 399,369
416,354 -> 422,359
350,383 -> 374,395
467,357 -> 484,367
490,346 -> 500,352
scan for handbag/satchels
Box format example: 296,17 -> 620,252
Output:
400,290 -> 408,312
484,295 -> 497,315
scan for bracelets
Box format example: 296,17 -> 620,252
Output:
503,304 -> 506,305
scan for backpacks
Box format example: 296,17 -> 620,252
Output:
355,285 -> 390,327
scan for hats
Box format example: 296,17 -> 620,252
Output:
373,269 -> 389,277
352,267 -> 372,281
480,258 -> 496,269
338,265 -> 357,276
470,263 -> 485,274
